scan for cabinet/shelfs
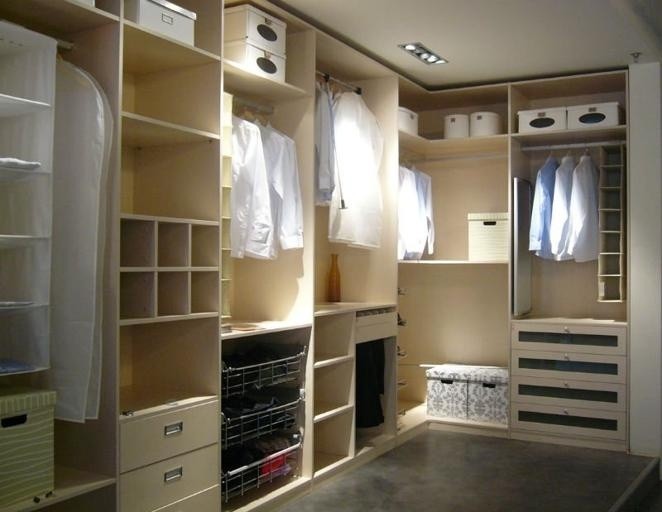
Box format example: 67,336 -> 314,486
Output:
0,0 -> 629,512
